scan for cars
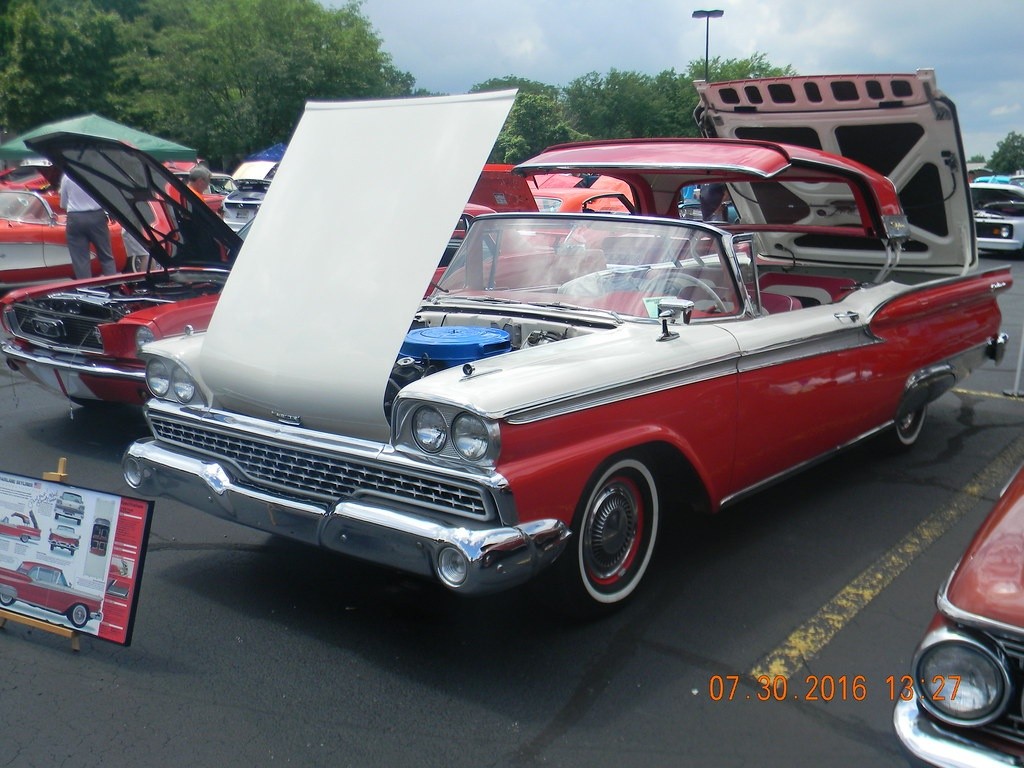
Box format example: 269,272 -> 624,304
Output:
0,561 -> 104,628
54,492 -> 85,525
0,510 -> 42,543
969,169 -> 1024,258
892,463 -> 1024,768
48,525 -> 81,556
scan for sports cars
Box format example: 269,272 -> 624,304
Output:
120,68 -> 1014,619
0,131 -> 737,406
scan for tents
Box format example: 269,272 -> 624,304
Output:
247,142 -> 288,162
0,115 -> 198,163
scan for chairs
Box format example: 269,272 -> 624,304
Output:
638,269 -> 859,315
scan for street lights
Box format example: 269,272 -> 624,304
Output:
692,10 -> 724,84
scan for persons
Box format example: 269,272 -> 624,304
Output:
967,170 -> 977,183
121,227 -> 156,272
185,164 -> 212,202
60,173 -> 116,280
681,185 -> 696,217
